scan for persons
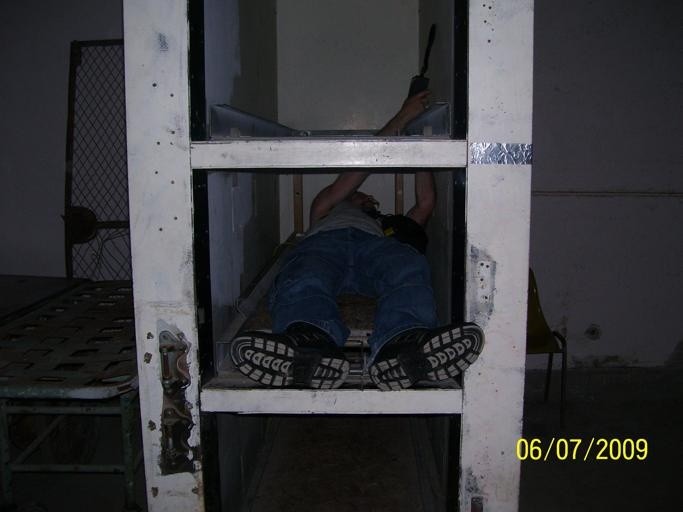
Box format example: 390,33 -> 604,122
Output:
229,90 -> 485,392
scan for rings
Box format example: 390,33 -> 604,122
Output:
425,104 -> 432,110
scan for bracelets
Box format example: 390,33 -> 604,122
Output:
386,111 -> 412,133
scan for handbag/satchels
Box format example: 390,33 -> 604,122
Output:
384,213 -> 429,251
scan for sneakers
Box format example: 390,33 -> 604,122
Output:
229,330 -> 350,389
370,323 -> 485,391
